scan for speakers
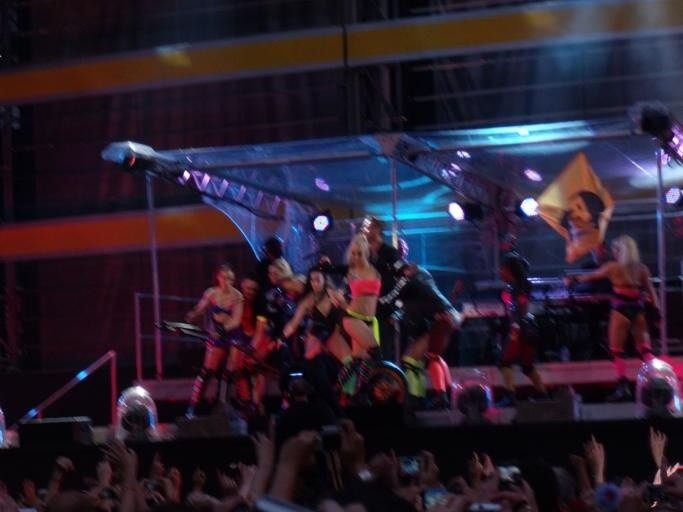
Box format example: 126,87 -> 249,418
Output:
18,417 -> 94,490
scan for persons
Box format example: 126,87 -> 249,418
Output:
533,165 -> 616,265
185,216 -> 465,416
0,418 -> 682,512
489,250 -> 553,399
563,232 -> 667,375
